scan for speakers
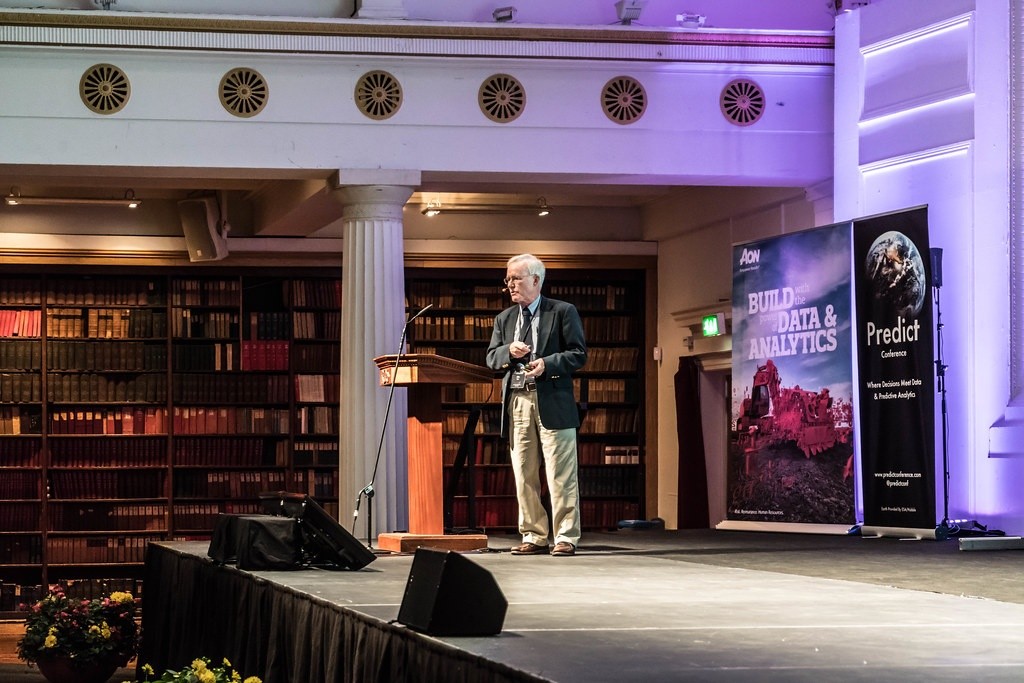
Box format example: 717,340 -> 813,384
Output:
397,545 -> 509,638
178,196 -> 228,261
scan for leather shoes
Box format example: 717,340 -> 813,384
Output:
551,542 -> 574,556
511,543 -> 550,554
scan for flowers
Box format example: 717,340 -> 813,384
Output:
15,583 -> 141,671
121,655 -> 263,683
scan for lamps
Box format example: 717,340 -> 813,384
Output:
492,6 -> 518,23
614,0 -> 642,25
422,199 -> 552,218
3,188 -> 142,209
676,10 -> 707,29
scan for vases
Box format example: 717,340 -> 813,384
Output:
38,653 -> 121,683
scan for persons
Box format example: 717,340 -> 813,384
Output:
486,253 -> 587,557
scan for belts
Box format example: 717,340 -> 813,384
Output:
509,383 -> 536,392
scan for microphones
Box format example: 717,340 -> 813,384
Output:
502,288 -> 508,292
405,303 -> 435,325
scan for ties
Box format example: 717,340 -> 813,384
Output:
517,307 -> 532,371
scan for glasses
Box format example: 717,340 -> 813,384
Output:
503,275 -> 533,285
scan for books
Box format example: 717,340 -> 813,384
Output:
173,535 -> 212,541
0,266 -> 167,337
0,535 -> 42,564
172,470 -> 286,528
294,470 -> 338,521
173,276 -> 339,339
295,405 -> 339,465
572,347 -> 643,403
0,403 -> 169,466
0,340 -> 169,402
405,285 -> 516,368
173,406 -> 288,465
549,286 -> 629,340
172,342 -> 239,402
442,379 -> 646,526
0,578 -> 143,611
48,469 -> 167,531
47,536 -> 168,565
0,469 -> 42,532
243,341 -> 340,403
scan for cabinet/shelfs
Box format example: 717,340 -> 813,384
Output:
0,252 -> 661,622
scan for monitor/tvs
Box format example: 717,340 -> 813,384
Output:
257,489 -> 378,573
